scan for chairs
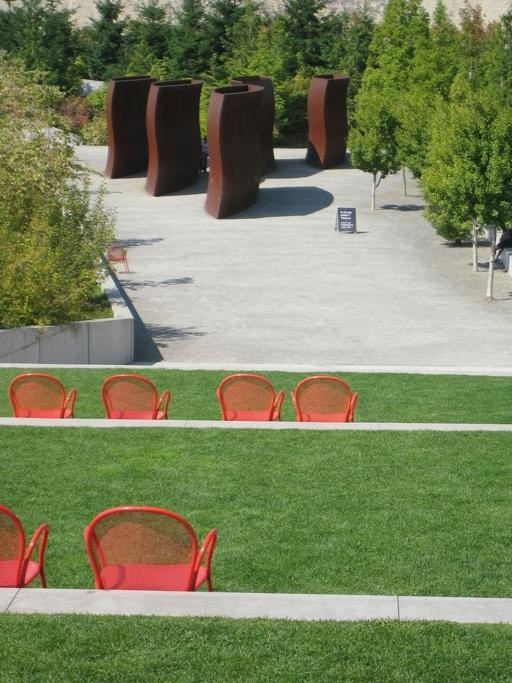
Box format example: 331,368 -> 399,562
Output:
289,375 -> 356,420
101,373 -> 170,420
0,503 -> 216,591
8,374 -> 78,419
107,246 -> 130,272
216,374 -> 286,419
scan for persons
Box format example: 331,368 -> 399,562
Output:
199,135 -> 209,173
